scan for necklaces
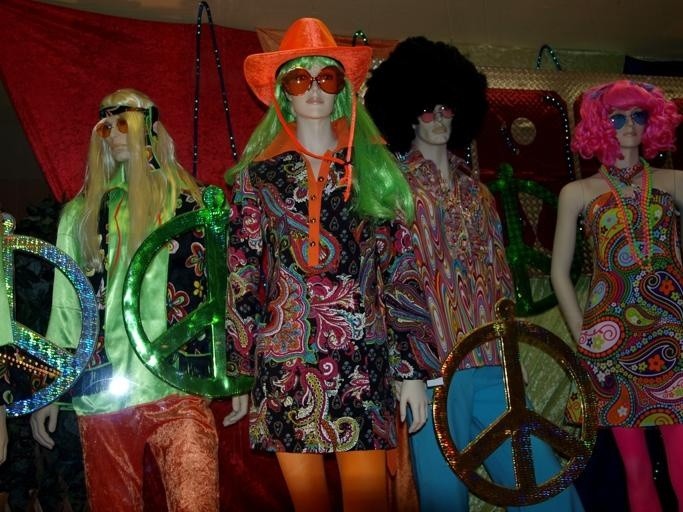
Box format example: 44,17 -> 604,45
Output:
597,157 -> 654,274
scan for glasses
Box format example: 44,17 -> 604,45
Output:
94,117 -> 132,138
608,109 -> 649,130
280,66 -> 347,96
417,105 -> 453,124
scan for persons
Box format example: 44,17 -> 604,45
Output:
365,36 -> 586,510
550,80 -> 683,511
0,231 -> 15,467
227,16 -> 445,511
30,88 -> 251,512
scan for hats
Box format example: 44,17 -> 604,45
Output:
240,16 -> 374,111
